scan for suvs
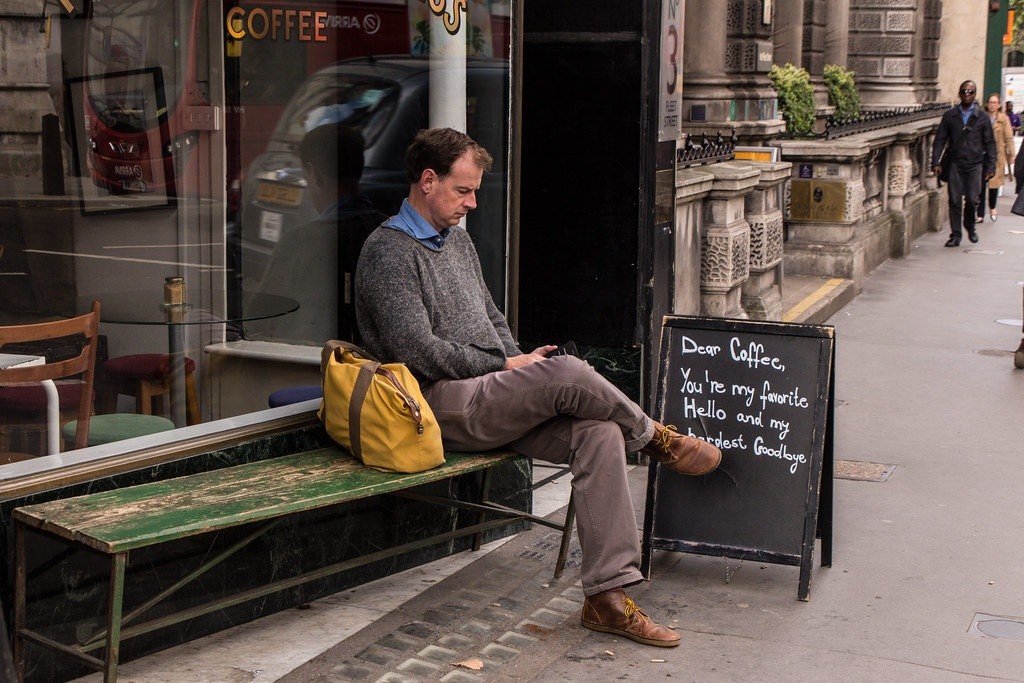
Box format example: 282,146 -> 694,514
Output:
217,53 -> 513,342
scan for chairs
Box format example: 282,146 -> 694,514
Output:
0,300 -> 100,465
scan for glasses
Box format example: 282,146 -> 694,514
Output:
961,90 -> 975,94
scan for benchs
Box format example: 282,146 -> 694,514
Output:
15,448 -> 575,683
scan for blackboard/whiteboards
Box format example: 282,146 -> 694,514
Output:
642,314 -> 835,566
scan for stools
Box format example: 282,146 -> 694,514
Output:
62,353 -> 201,451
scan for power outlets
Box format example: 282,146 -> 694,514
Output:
188,80 -> 209,105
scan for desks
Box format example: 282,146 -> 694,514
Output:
53,288 -> 301,429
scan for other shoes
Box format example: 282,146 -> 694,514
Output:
975,218 -> 983,223
967,229 -> 978,242
990,209 -> 997,221
945,235 -> 961,247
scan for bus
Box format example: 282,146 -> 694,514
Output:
36,0 -> 511,201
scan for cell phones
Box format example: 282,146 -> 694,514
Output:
543,340 -> 580,360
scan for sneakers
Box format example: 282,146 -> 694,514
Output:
639,420 -> 722,475
581,586 -> 681,646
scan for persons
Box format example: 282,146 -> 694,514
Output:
931,80 -> 997,247
353,126 -> 723,647
975,93 -> 1024,224
243,123 -> 400,347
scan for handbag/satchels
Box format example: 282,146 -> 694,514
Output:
939,150 -> 952,181
318,340 -> 446,472
